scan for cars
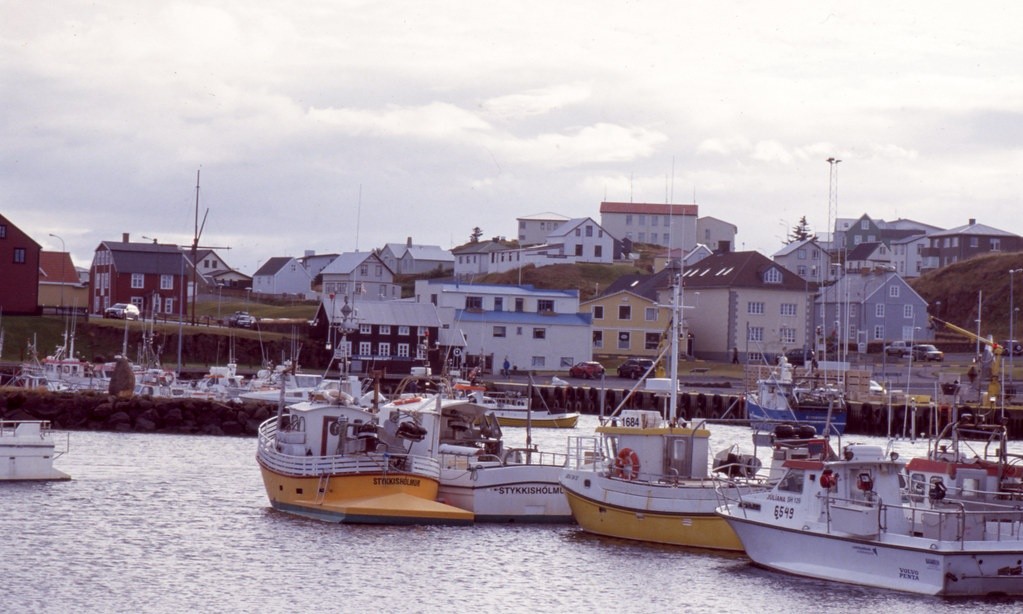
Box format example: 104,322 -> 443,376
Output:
105,303 -> 140,320
871,380 -> 887,394
911,345 -> 944,363
776,349 -> 815,366
229,315 -> 254,328
569,362 -> 605,379
617,359 -> 656,380
998,340 -> 1023,356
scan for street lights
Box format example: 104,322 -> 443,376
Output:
246,287 -> 251,312
49,233 -> 65,305
1009,270 -> 1014,384
143,236 -> 159,312
877,265 -> 896,402
218,284 -> 224,320
936,301 -> 942,318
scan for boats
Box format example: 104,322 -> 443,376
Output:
743,364 -> 847,436
256,402 -> 475,526
42,356 -> 390,412
558,411 -> 768,551
0,420 -> 71,480
709,425 -> 1023,597
440,384 -> 580,429
377,393 -> 574,524
896,421 -> 1023,522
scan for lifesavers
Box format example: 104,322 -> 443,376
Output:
164,372 -> 174,387
616,448 -> 639,482
392,395 -> 423,406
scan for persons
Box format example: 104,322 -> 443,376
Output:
409,379 -> 418,393
502,357 -> 511,377
74,350 -> 82,360
731,347 -> 740,364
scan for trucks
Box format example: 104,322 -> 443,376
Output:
885,341 -> 911,358
